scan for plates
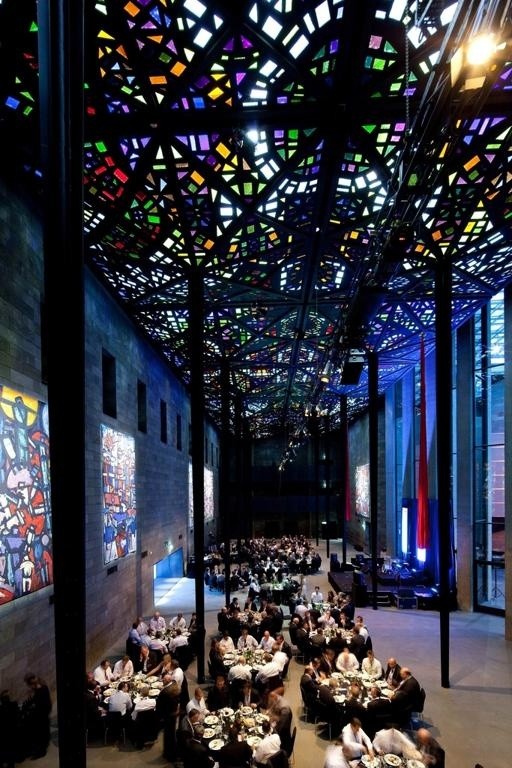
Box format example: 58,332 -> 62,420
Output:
103,673 -> 164,704
201,706 -> 274,750
320,670 -> 394,706
360,754 -> 425,768
223,648 -> 274,671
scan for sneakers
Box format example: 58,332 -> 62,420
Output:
30,750 -> 47,760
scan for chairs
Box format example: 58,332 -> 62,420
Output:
289,626 -> 375,664
124,626 -> 205,672
215,608 -> 285,637
210,646 -> 290,696
174,703 -> 298,768
86,670 -> 186,751
300,665 -> 427,745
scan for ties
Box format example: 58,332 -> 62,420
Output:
354,733 -> 362,756
105,670 -> 108,680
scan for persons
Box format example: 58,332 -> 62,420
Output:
129,610 -> 196,656
288,585 -> 369,656
204,534 -> 316,597
86,646 -> 184,726
182,676 -> 293,767
301,648 -> 421,731
214,596 -> 291,684
325,716 -> 445,768
20,671 -> 53,761
